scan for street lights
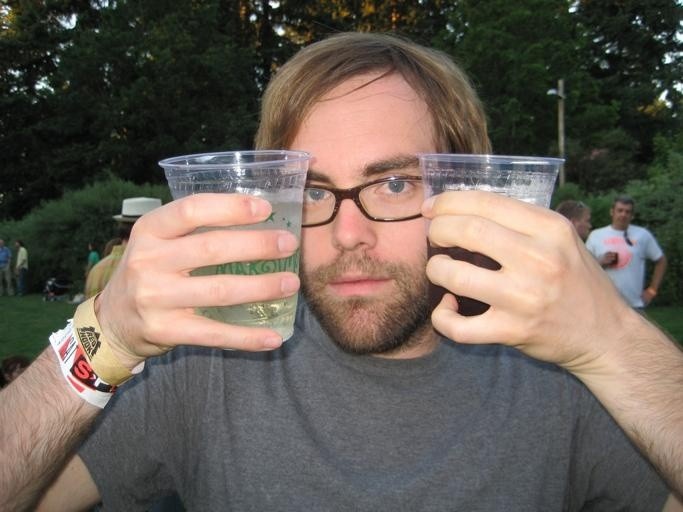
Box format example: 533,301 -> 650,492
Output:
545,77 -> 567,185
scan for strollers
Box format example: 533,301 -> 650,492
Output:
43,277 -> 75,303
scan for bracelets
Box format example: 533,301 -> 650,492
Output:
48,315 -> 112,411
74,292 -> 147,386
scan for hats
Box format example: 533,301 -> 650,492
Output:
111,197 -> 161,222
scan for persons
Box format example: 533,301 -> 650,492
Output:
0,26 -> 683,512
585,193 -> 668,315
646,285 -> 658,296
84,195 -> 163,294
12,239 -> 29,297
84,240 -> 99,272
555,197 -> 591,240
0,237 -> 14,296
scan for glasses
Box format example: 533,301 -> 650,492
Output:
624,227 -> 633,246
572,200 -> 585,215
300,177 -> 426,228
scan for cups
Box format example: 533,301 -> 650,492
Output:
413,150 -> 566,337
156,148 -> 313,355
607,248 -> 619,264
